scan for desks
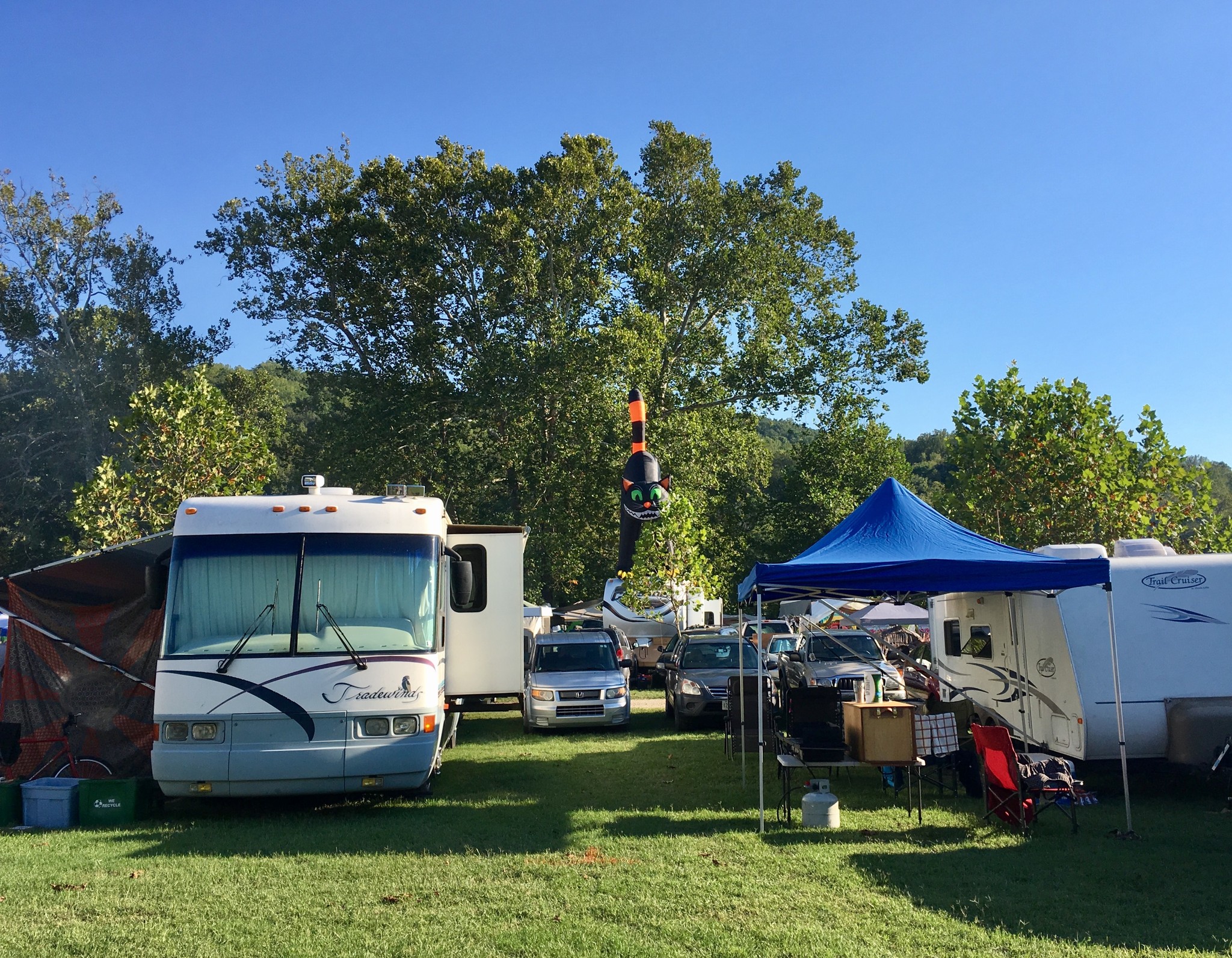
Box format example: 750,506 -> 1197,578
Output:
777,754 -> 925,826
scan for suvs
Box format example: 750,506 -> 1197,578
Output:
665,634 -> 779,728
902,641 -> 931,691
568,624 -> 639,689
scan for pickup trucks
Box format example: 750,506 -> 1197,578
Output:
777,629 -> 907,702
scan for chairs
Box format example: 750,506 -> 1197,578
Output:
881,698 -> 1084,837
726,648 -> 740,667
723,675 -> 852,785
686,649 -> 705,668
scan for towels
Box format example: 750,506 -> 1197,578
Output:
915,714 -> 931,758
929,712 -> 960,758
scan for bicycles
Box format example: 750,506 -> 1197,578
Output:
0,712 -> 114,782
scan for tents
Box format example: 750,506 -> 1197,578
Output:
739,478 -> 1132,838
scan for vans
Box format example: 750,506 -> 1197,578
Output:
742,620 -> 793,641
655,624 -> 739,677
519,632 -> 633,734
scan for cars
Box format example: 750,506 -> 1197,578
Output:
762,634 -> 800,682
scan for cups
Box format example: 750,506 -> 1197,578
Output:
853,680 -> 864,703
864,671 -> 883,703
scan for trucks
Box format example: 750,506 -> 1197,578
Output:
552,577 -> 723,681
928,539 -> 1232,761
0,474 -> 532,801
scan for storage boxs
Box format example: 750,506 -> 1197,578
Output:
78,776 -> 153,827
0,779 -> 30,827
20,777 -> 89,828
794,700 -> 918,763
